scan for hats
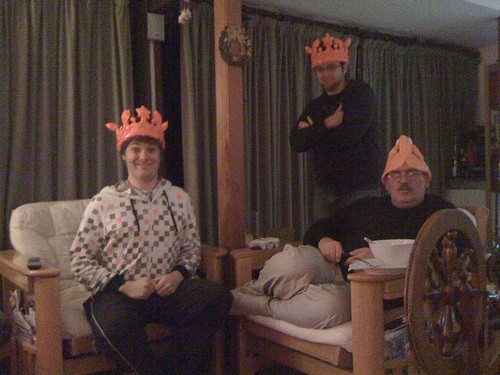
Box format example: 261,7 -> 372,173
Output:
381,136 -> 432,183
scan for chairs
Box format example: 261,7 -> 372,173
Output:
0,196 -> 228,375
227,207 -> 491,375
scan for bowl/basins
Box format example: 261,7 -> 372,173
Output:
368,239 -> 415,268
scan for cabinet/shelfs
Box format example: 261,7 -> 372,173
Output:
450,123 -> 498,176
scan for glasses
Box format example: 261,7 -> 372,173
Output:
316,65 -> 342,73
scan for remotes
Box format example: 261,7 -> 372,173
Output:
27,256 -> 41,271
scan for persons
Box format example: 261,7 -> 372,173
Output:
228,134 -> 458,330
69,106 -> 234,375
290,32 -> 380,224
